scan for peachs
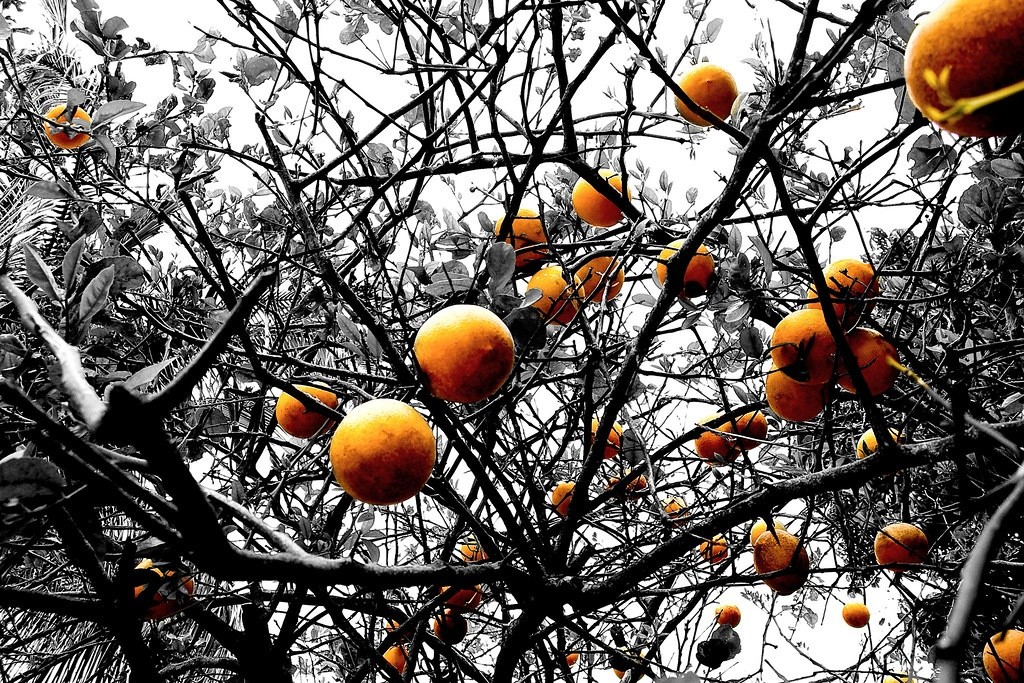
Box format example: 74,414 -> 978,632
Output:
41,1 -> 1024,683
